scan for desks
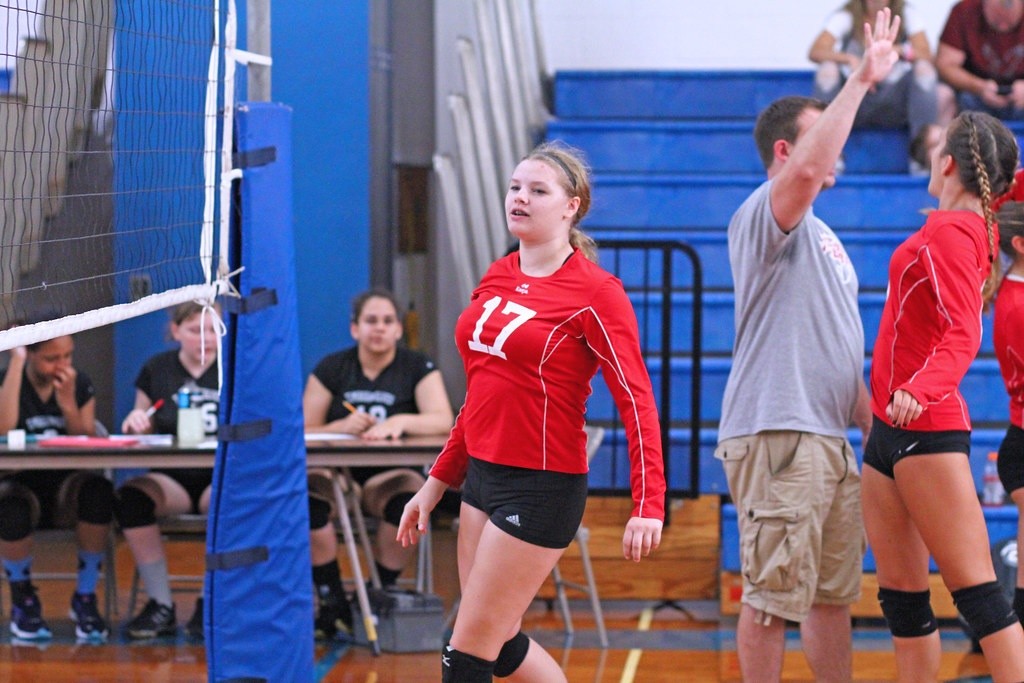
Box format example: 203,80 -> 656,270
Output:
0,432 -> 453,654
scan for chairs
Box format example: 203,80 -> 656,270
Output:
441,425 -> 608,649
0,419 -> 119,633
122,513 -> 207,633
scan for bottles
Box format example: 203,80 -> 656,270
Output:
983,452 -> 1004,507
176,378 -> 205,448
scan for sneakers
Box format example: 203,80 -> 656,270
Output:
120,594 -> 177,639
185,594 -> 205,637
9,589 -> 53,638
67,593 -> 110,639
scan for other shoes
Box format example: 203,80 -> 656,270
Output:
835,154 -> 845,173
311,589 -> 352,638
908,150 -> 931,178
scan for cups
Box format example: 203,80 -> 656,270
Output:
8,428 -> 26,450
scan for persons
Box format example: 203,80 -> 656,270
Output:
302,288 -> 456,641
809,0 -> 938,177
859,111 -> 1024,683
121,299 -> 224,638
393,139 -> 667,683
0,319 -> 113,642
712,7 -> 902,683
936,0 -> 1024,120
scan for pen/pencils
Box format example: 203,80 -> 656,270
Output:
127,398 -> 166,435
342,400 -> 374,424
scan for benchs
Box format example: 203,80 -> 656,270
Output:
545,69 -> 1024,495
721,502 -> 1019,619
